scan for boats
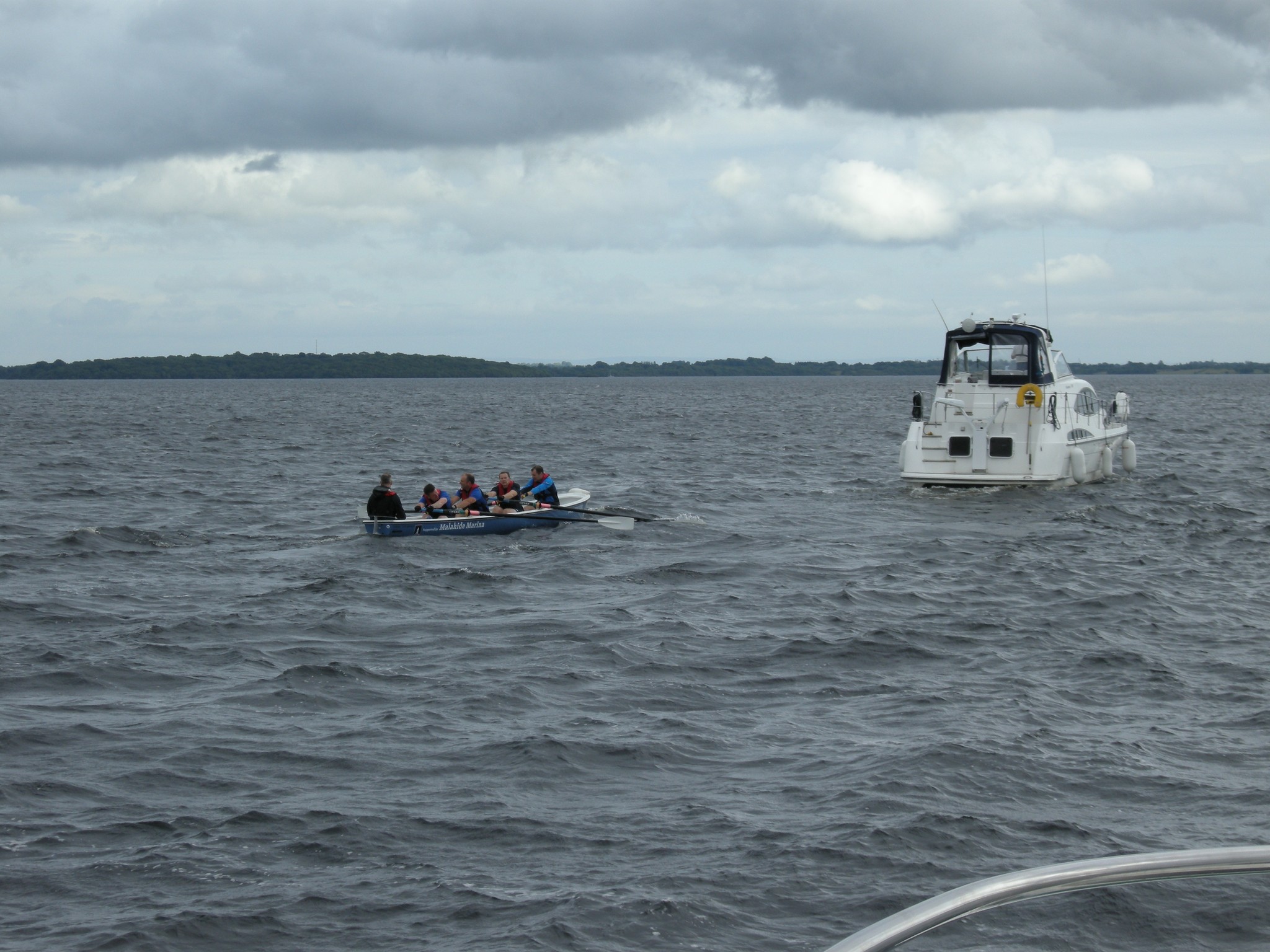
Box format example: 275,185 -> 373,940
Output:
362,487 -> 591,537
899,227 -> 1138,488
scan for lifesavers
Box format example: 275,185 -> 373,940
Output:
1016,383 -> 1043,408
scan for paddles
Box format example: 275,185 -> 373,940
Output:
485,497 -> 655,523
405,508 -> 635,530
